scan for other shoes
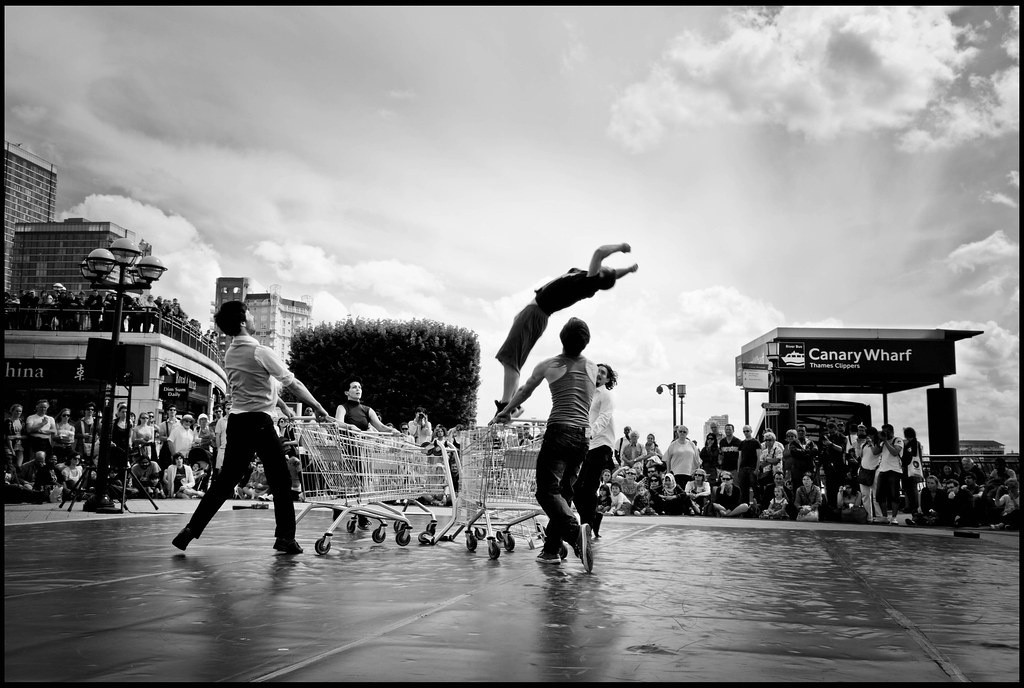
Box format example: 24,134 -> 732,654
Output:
604,512 -> 614,516
891,517 -> 899,524
171,528 -> 194,551
874,517 -> 890,524
358,518 -> 369,530
258,494 -> 273,500
593,512 -> 603,537
273,539 -> 303,553
488,400 -> 524,425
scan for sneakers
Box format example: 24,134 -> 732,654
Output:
574,523 -> 594,572
535,548 -> 562,564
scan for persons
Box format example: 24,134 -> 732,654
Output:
335,376 -> 407,439
3,288 -> 1019,527
572,364 -> 615,537
171,300 -> 328,552
492,317 -> 599,573
493,243 -> 637,423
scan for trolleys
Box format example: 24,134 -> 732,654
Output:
280,414 -> 548,559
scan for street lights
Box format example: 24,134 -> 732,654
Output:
677,384 -> 687,425
80,238 -> 169,511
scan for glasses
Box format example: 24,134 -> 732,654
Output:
679,424 -> 845,442
141,409 -> 176,419
73,458 -> 80,461
650,479 -> 658,482
307,410 -> 311,412
86,408 -> 94,411
281,421 -> 283,423
401,427 -> 408,430
61,414 -> 70,417
694,474 -> 703,477
723,477 -> 732,480
215,408 -> 230,413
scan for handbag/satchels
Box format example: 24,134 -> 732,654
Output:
907,456 -> 924,478
857,466 -> 875,487
848,504 -> 867,524
159,440 -> 172,469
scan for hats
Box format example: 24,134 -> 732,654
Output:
181,415 -> 195,424
857,421 -> 867,429
198,414 -> 208,423
